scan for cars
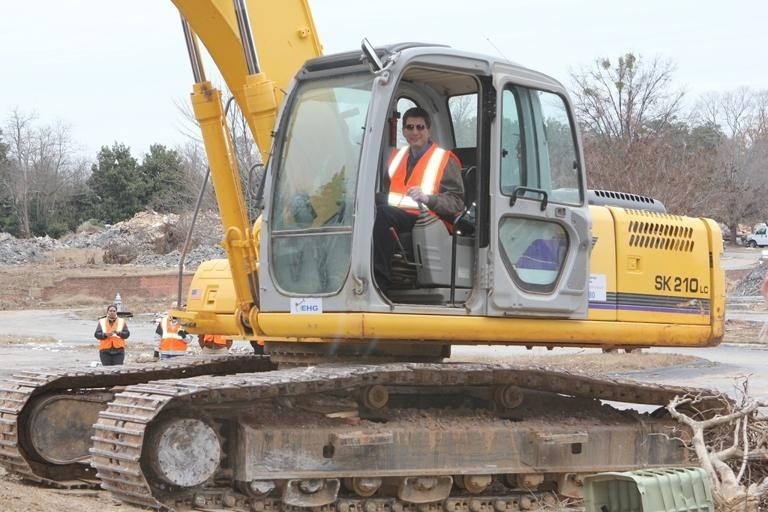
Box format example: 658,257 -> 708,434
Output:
720,222 -> 768,248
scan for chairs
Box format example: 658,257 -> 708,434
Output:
393,146 -> 483,296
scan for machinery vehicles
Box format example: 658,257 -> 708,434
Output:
0,0 -> 766,512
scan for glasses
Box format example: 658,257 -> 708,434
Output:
403,124 -> 425,130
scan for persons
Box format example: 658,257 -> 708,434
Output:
153,301 -> 234,359
373,107 -> 465,295
95,305 -> 130,366
